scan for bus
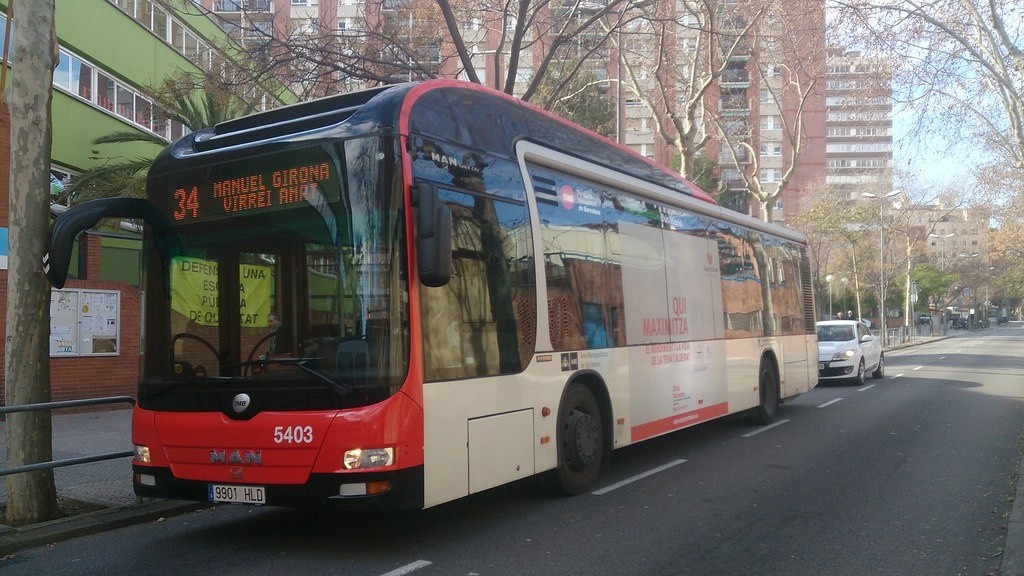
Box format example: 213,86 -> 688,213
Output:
42,78 -> 822,517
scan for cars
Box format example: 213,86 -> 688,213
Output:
816,319 -> 886,386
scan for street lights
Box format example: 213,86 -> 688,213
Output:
927,233 -> 957,336
861,189 -> 902,342
824,274 -> 834,321
840,277 -> 850,319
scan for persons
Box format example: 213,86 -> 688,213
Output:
428,286 -> 461,366
260,313 -> 280,361
836,312 -> 844,320
847,310 -> 856,320
